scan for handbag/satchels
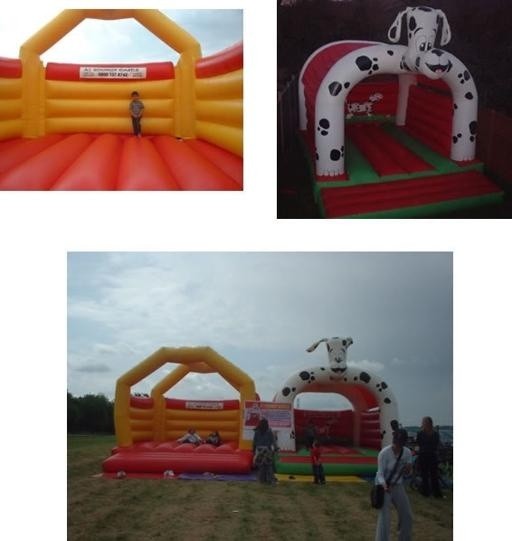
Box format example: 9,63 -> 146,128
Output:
371,484 -> 385,508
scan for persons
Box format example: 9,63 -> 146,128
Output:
204,430 -> 223,445
310,440 -> 327,485
251,417 -> 280,483
414,417 -> 446,499
129,91 -> 144,138
374,429 -> 414,541
177,427 -> 202,447
390,420 -> 399,444
303,421 -> 317,451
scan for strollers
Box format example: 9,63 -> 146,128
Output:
410,460 -> 449,492
